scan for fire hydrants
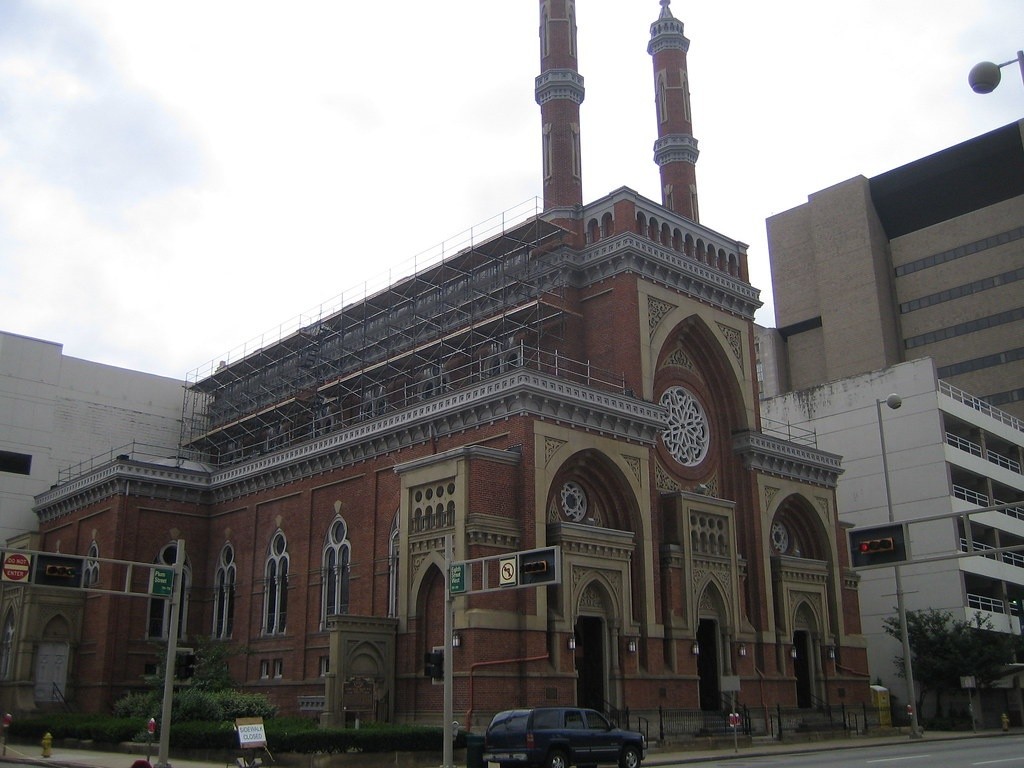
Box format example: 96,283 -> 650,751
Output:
1001,713 -> 1009,731
41,732 -> 52,759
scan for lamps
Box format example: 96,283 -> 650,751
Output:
738,642 -> 747,656
789,646 -> 796,658
691,641 -> 700,655
567,636 -> 575,650
452,631 -> 461,647
626,639 -> 637,653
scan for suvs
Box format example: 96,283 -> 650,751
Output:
482,708 -> 646,768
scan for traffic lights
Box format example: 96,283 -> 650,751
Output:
859,538 -> 893,555
423,651 -> 443,680
523,560 -> 548,574
177,650 -> 196,680
45,565 -> 77,579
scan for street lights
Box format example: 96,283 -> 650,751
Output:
968,50 -> 1024,94
875,393 -> 922,737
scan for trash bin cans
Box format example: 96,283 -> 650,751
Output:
466,735 -> 488,768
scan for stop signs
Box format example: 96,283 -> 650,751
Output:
1,552 -> 32,583
